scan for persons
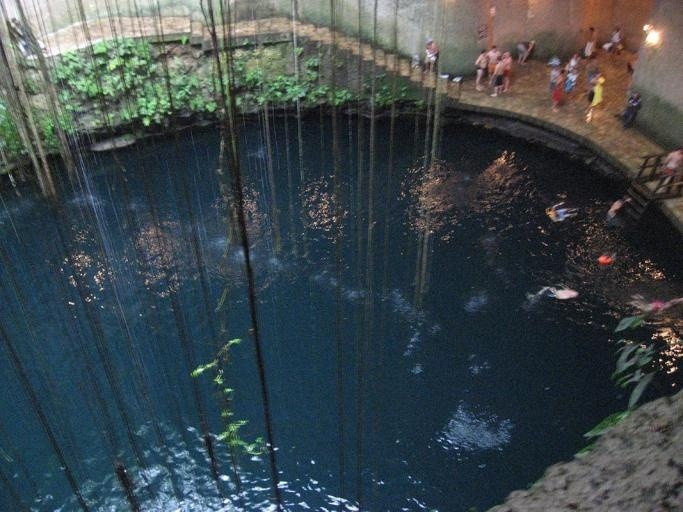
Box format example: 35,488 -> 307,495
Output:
656,146 -> 683,193
423,38 -> 439,73
545,202 -> 578,222
608,197 -> 632,218
475,25 -> 642,128
525,284 -> 579,302
